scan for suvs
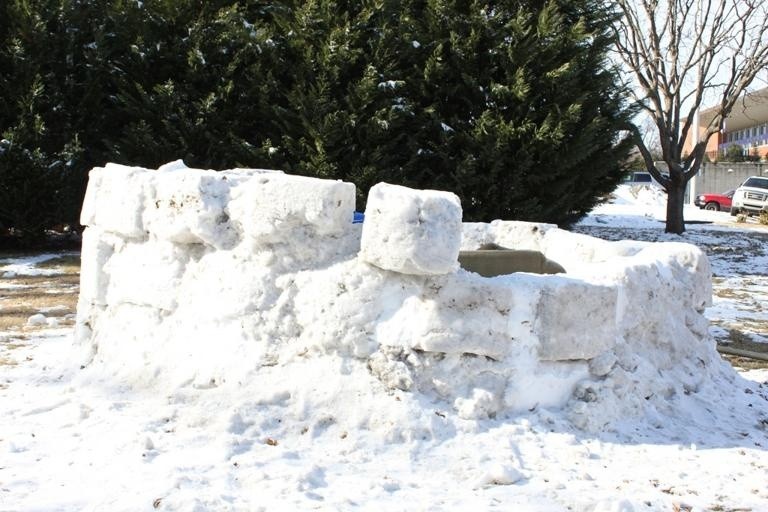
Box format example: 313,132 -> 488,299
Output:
731,176 -> 768,218
623,172 -> 669,192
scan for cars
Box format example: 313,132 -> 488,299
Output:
694,189 -> 735,211
